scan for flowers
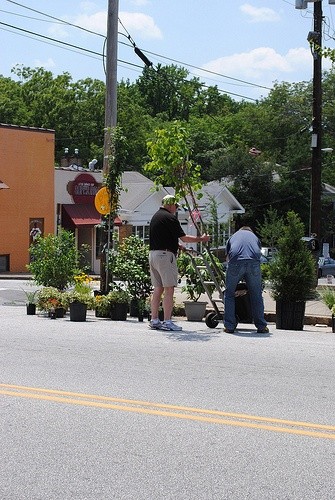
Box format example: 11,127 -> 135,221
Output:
25,283 -> 129,308
148,290 -> 175,312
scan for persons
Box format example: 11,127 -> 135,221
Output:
149,195 -> 209,331
224,230 -> 269,334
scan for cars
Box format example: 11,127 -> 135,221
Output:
317,257 -> 335,279
260,248 -> 278,266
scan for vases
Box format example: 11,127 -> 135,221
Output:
149,308 -> 172,321
26,303 -> 132,322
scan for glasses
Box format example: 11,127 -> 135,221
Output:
175,204 -> 178,209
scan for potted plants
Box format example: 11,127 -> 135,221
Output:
108,236 -> 153,322
262,213 -> 317,331
176,252 -> 222,321
319,287 -> 335,332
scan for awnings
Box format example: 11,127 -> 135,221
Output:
62,204 -> 122,228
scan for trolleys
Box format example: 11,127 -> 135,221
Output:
182,247 -> 238,329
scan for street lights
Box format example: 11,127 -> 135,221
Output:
309,147 -> 333,237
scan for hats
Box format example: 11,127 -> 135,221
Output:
162,195 -> 180,206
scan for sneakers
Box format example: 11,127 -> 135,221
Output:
150,320 -> 163,329
226,327 -> 234,333
257,326 -> 269,333
161,322 -> 182,330
